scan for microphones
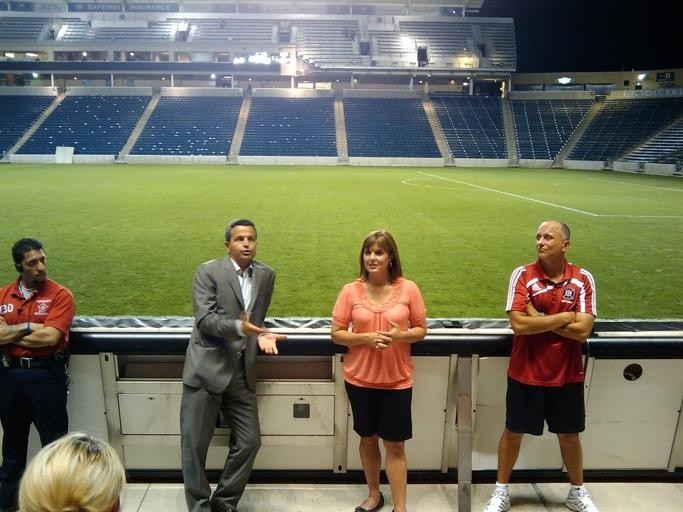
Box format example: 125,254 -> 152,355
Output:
23,271 -> 37,282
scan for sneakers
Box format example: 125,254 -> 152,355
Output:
564,486 -> 600,512
481,484 -> 512,512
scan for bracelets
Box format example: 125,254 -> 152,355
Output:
25,319 -> 30,334
537,312 -> 547,317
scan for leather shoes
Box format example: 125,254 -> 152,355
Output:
391,509 -> 394,512
354,490 -> 385,512
1,351 -> 66,370
209,501 -> 239,512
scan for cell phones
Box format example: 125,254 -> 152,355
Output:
442,321 -> 462,328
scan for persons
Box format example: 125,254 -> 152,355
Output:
0,237 -> 76,512
16,431 -> 128,512
331,231 -> 428,512
477,219 -> 599,512
180,218 -> 287,511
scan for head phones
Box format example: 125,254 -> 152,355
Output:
15,264 -> 24,273
